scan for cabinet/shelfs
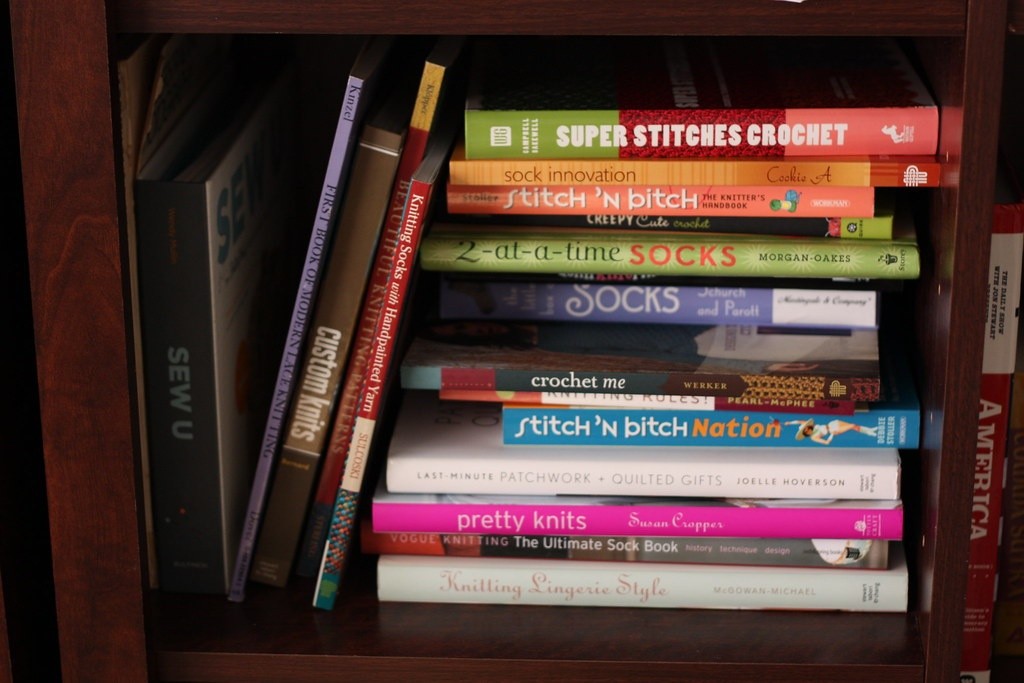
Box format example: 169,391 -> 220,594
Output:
6,0 -> 1010,683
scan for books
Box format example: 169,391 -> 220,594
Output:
958,200 -> 1024,683
112,36 -> 466,612
353,38 -> 944,612
